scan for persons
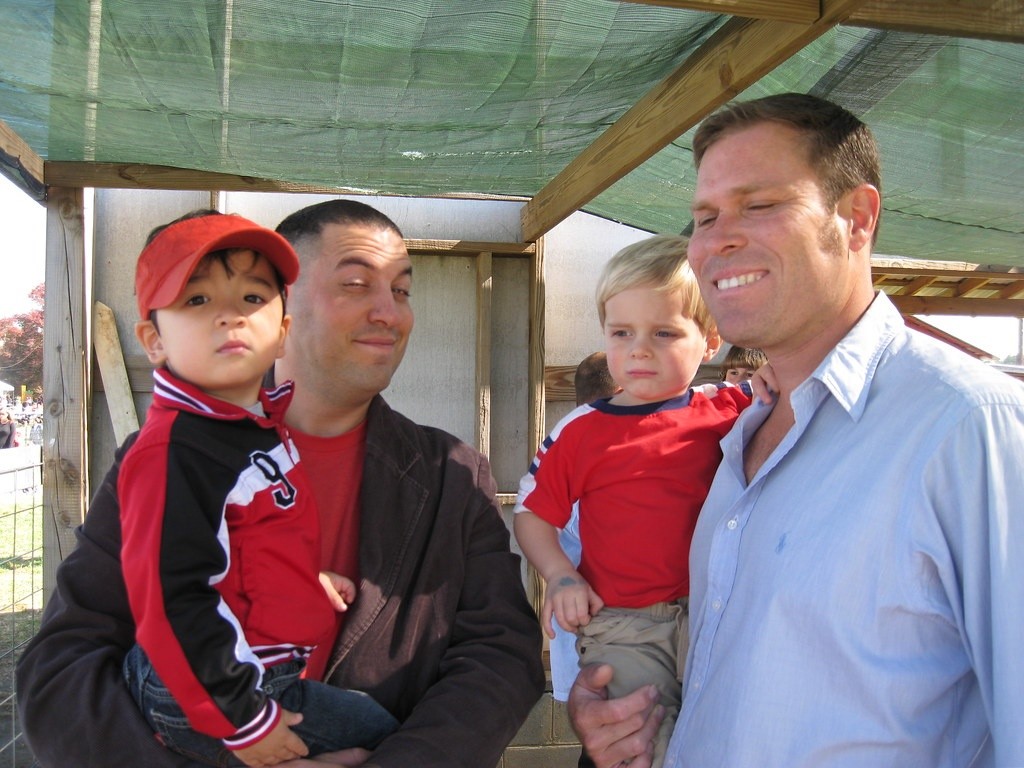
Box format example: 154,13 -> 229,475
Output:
0,393 -> 43,450
118,209 -> 397,768
12,199 -> 545,768
567,90 -> 1024,768
512,234 -> 779,768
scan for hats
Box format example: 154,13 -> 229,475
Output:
136,214 -> 301,321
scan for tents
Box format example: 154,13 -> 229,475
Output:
0,380 -> 15,407
